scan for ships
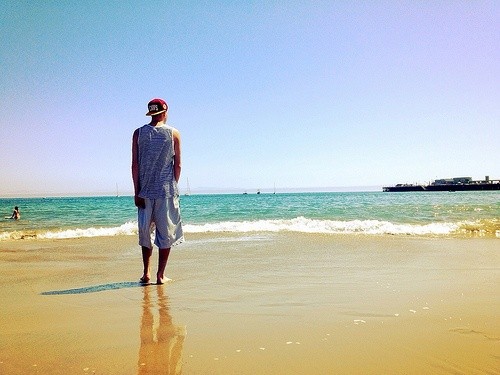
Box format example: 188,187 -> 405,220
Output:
382,175 -> 500,192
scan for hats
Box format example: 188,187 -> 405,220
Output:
146,98 -> 168,116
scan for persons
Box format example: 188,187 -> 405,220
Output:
132,99 -> 185,285
11,206 -> 20,219
